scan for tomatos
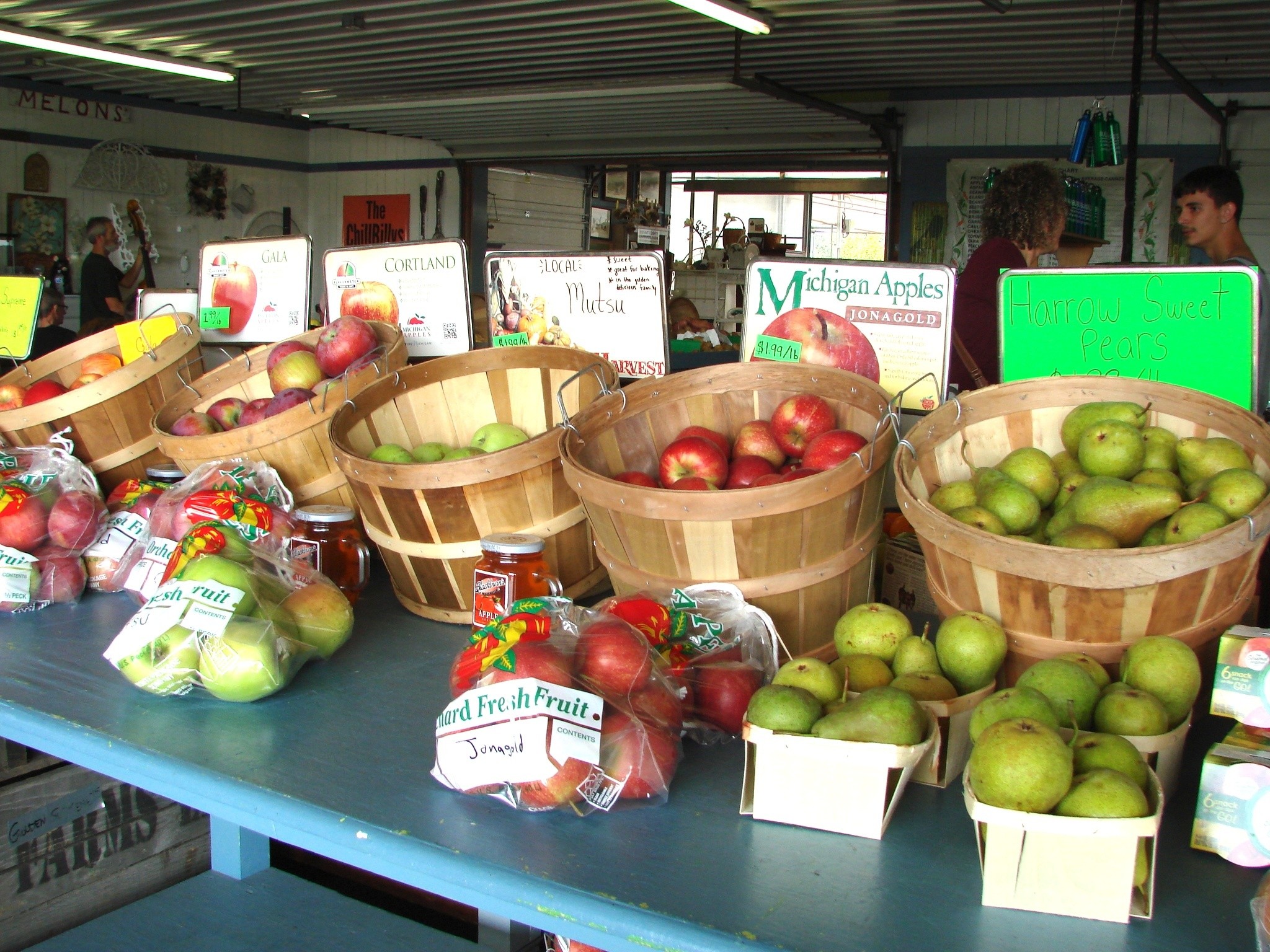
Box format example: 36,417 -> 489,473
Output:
883,512 -> 915,539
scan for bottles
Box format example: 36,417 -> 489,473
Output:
1067,100 -> 1124,168
987,166 -> 1001,190
1060,172 -> 1105,239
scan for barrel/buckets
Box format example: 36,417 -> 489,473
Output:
147,319 -> 411,551
0,302 -> 205,506
765,232 -> 782,250
723,217 -> 745,249
326,345 -> 625,624
886,372 -> 1270,692
557,363 -> 897,670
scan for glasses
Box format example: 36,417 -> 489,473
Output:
56,304 -> 68,310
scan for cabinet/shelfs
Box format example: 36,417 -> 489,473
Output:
0,586 -> 1259,951
672,269 -> 745,334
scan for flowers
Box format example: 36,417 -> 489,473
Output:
683,213 -> 737,265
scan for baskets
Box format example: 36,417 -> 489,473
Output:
963,748 -> 1164,926
739,705 -> 942,843
1056,708 -> 1195,802
836,676 -> 997,788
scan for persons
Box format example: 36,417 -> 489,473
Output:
947,161 -> 1069,392
78,217 -> 151,341
1173,164 -> 1270,420
78,317 -> 124,340
16,287 -> 77,366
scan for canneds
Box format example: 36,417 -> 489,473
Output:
146,464 -> 187,484
291,504 -> 370,604
472,532 -> 563,635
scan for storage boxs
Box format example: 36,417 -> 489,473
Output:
881,533 -> 936,614
1189,625 -> 1270,869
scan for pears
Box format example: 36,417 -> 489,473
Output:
928,401 -> 1269,550
969,635 -> 1200,893
745,602 -> 1007,746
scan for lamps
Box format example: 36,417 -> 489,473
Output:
0,22 -> 238,83
670,0 -> 776,35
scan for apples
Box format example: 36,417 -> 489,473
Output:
466,598 -> 763,819
368,422 -> 528,463
340,281 -> 399,325
0,453 -> 294,611
491,297 -> 519,337
750,308 -> 880,385
89,498 -> 355,702
171,317 -> 380,436
212,261 -> 258,335
611,393 -> 866,490
0,353 -> 122,410
1238,637 -> 1270,667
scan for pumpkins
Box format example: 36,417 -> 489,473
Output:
516,314 -> 548,345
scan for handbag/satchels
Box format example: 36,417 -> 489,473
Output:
116,458 -> 295,605
102,520 -> 353,700
1,427 -> 110,611
431,576 -> 686,816
86,479 -> 175,594
641,580 -> 795,748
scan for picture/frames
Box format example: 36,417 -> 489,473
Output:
7,194 -> 67,270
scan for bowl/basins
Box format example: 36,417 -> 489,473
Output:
779,244 -> 797,254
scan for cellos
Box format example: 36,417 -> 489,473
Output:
127,199 -> 156,289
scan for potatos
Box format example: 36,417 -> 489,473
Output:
670,316 -> 740,353
